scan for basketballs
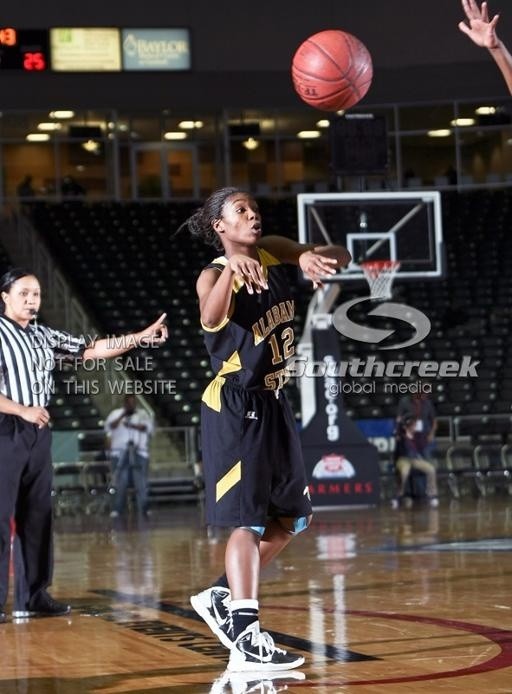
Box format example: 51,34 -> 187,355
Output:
295,29 -> 374,110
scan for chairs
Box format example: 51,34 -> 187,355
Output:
378,440 -> 512,499
52,463 -> 112,517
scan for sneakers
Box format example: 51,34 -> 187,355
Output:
209,667 -> 306,694
228,634 -> 304,672
189,586 -> 234,648
11,620 -> 74,644
12,591 -> 70,619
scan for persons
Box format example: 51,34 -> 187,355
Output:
0,268 -> 170,623
16,173 -> 34,211
173,186 -> 351,671
458,0 -> 512,97
397,381 -> 438,463
389,413 -> 440,510
103,394 -> 155,518
